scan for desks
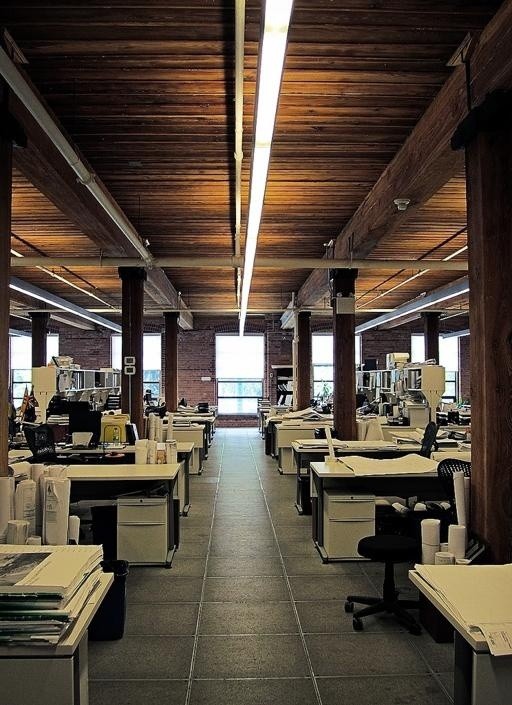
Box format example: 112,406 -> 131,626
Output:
408,560 -> 512,704
15,406 -> 217,568
261,404 -> 474,565
0,571 -> 117,705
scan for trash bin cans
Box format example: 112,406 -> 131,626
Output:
89,560 -> 129,641
298,475 -> 313,515
315,428 -> 334,438
264,425 -> 277,455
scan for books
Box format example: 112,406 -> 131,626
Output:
282,421 -> 302,429
379,403 -> 397,416
46,414 -> 69,425
173,420 -> 191,427
0,545 -> 104,647
357,370 -> 421,390
414,563 -> 512,656
295,439 -> 397,450
258,381 -> 293,407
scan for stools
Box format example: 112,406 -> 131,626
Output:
340,536 -> 425,639
